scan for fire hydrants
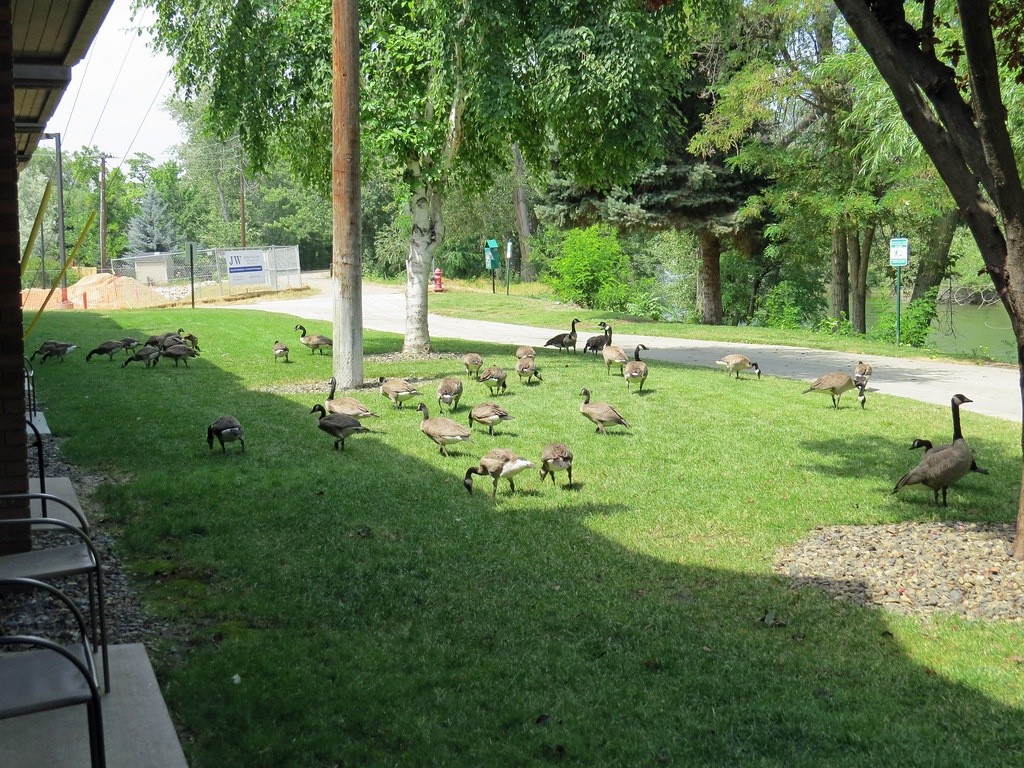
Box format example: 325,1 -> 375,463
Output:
430,267 -> 443,292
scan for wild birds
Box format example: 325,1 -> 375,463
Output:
310,377 -> 380,452
30,340 -> 79,364
544,319 -> 581,353
578,389 -> 628,433
416,403 -> 471,457
294,324 -> 333,355
206,415 -> 244,453
716,354 -> 761,379
802,360 -> 872,408
379,376 -> 463,413
463,352 -> 507,395
468,402 -> 515,436
86,328 -> 200,369
539,443 -> 573,486
584,322 -> 628,376
463,448 -> 535,495
274,341 -> 289,362
891,394 -> 989,506
624,344 -> 650,392
516,347 -> 543,383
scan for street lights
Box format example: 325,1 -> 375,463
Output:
39,133 -> 68,303
